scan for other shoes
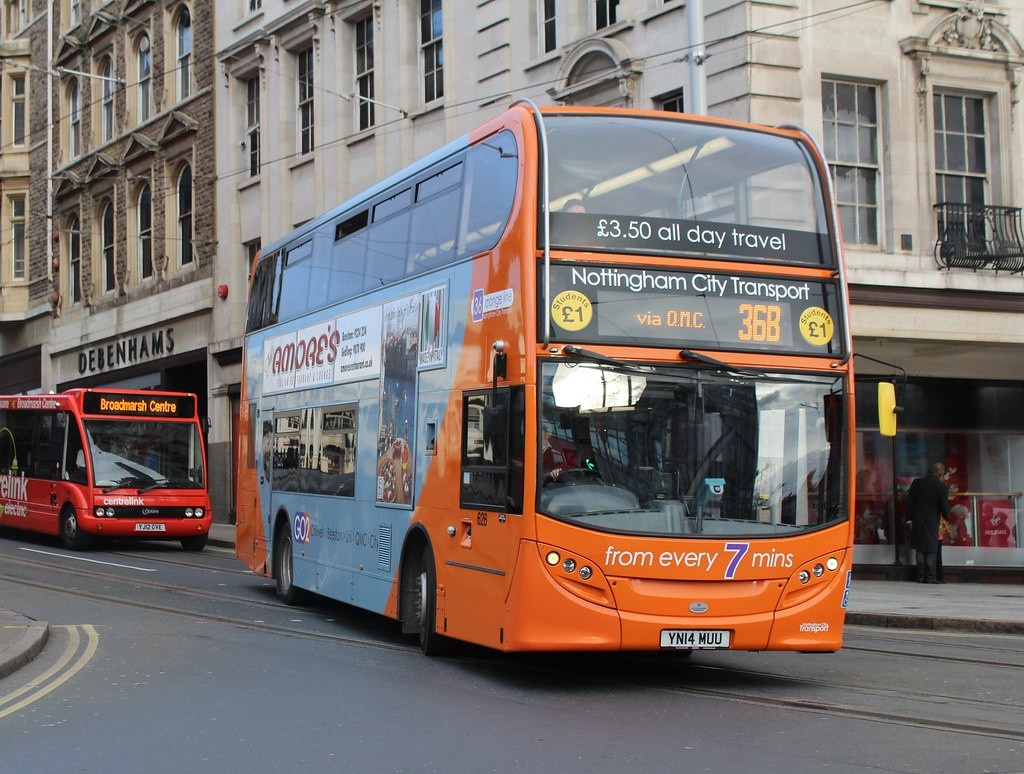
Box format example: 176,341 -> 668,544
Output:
925,579 -> 942,584
919,578 -> 928,583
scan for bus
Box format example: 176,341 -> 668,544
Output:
0,387 -> 214,551
235,97 -> 908,659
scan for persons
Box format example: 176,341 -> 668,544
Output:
517,417 -> 577,493
982,502 -> 1010,547
76,432 -> 100,468
906,462 -> 949,584
